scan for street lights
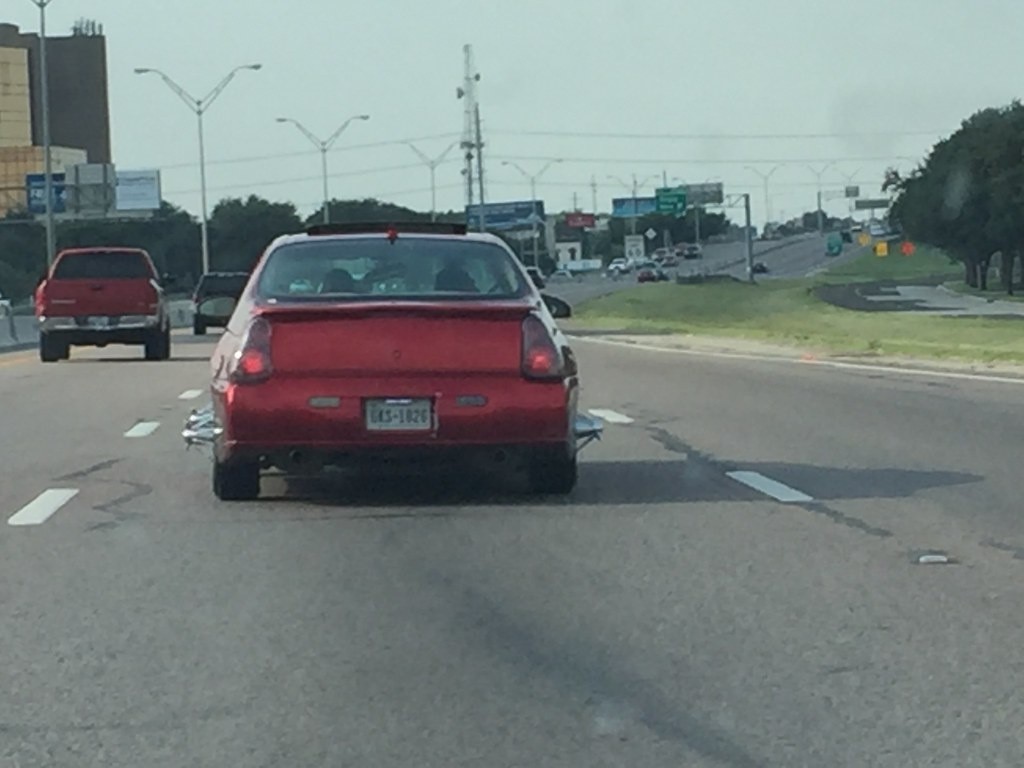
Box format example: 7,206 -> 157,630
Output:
276,115 -> 371,224
501,159 -> 563,269
132,63 -> 262,272
400,140 -> 461,224
605,173 -> 660,235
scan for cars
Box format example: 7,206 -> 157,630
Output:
32,246 -> 172,363
752,262 -> 770,275
185,221 -> 606,504
607,241 -> 704,283
193,272 -> 252,335
823,218 -> 883,259
